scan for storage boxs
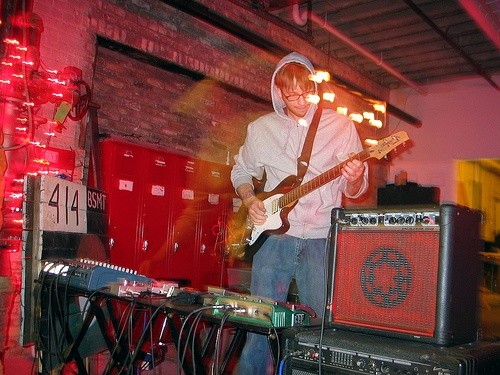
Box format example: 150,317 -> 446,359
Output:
227,267 -> 252,293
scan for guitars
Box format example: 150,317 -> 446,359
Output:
221,131 -> 410,262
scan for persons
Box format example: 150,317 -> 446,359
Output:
230,53 -> 369,375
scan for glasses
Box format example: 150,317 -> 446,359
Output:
281,90 -> 315,101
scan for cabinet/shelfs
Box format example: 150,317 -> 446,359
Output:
88,135 -> 252,345
